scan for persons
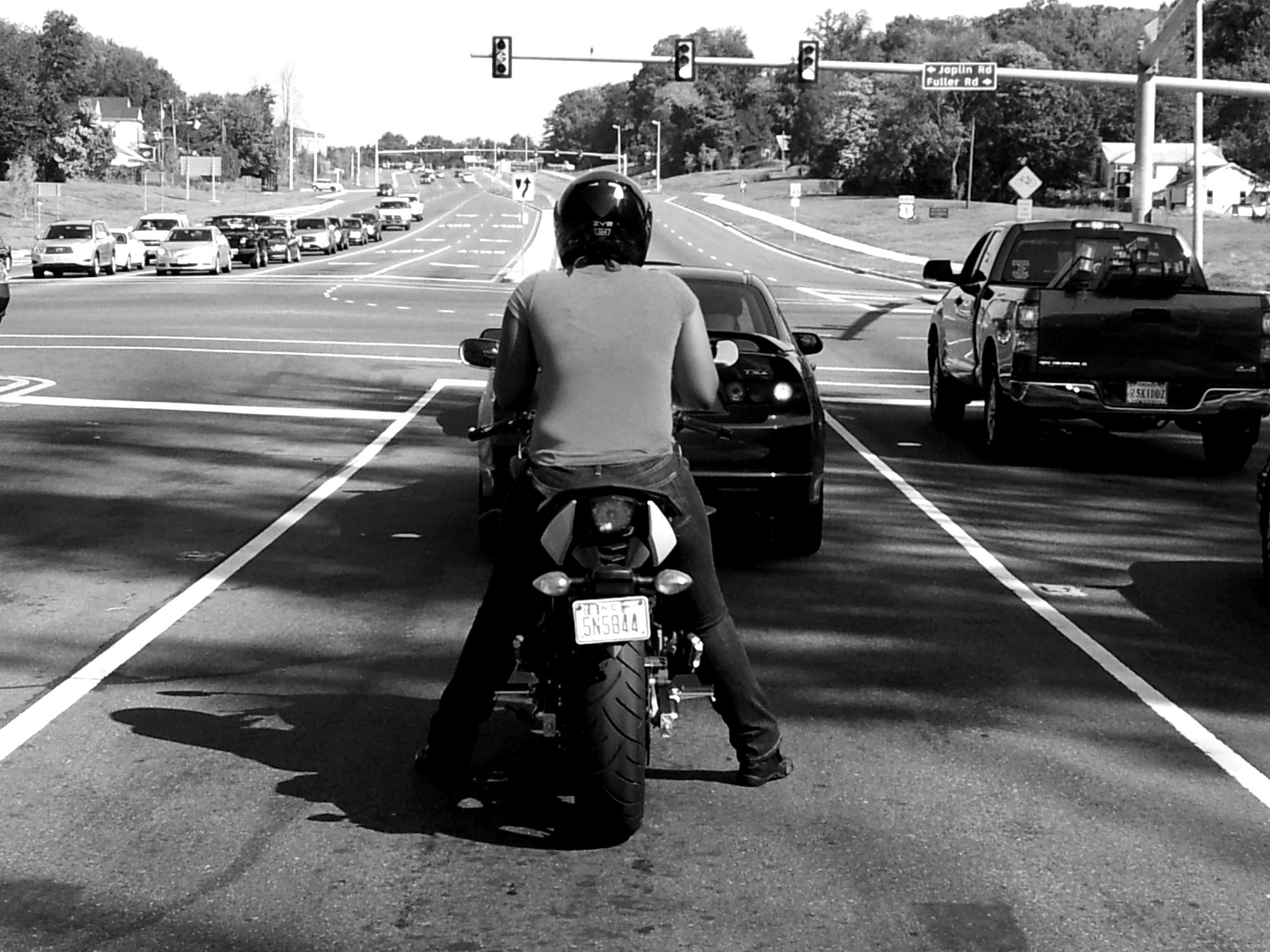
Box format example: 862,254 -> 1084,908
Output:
407,170 -> 794,787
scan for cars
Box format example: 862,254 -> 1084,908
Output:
311,178 -> 343,193
412,163 -> 475,183
0,236 -> 12,273
350,210 -> 384,242
377,183 -> 396,197
466,261 -> 834,560
295,215 -> 340,256
207,213 -> 303,269
154,226 -> 232,276
329,216 -> 352,251
108,227 -> 148,272
343,218 -> 370,245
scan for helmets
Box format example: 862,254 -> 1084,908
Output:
554,172 -> 653,269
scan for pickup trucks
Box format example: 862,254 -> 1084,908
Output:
920,217 -> 1270,457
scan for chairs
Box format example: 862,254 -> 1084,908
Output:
697,291 -> 743,331
1012,242 -> 1059,284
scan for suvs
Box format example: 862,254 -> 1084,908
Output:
131,213 -> 191,266
399,193 -> 426,221
30,218 -> 118,277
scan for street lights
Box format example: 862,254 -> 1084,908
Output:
652,119 -> 662,192
612,123 -> 622,173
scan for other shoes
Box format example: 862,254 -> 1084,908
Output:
413,747 -> 456,788
739,755 -> 793,787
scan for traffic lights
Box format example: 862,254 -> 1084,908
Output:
673,39 -> 696,81
491,35 -> 514,79
798,39 -> 819,84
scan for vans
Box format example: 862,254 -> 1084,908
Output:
375,198 -> 413,231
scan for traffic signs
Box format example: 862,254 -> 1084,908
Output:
921,60 -> 998,91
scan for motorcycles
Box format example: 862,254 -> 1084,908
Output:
453,329 -> 767,844
